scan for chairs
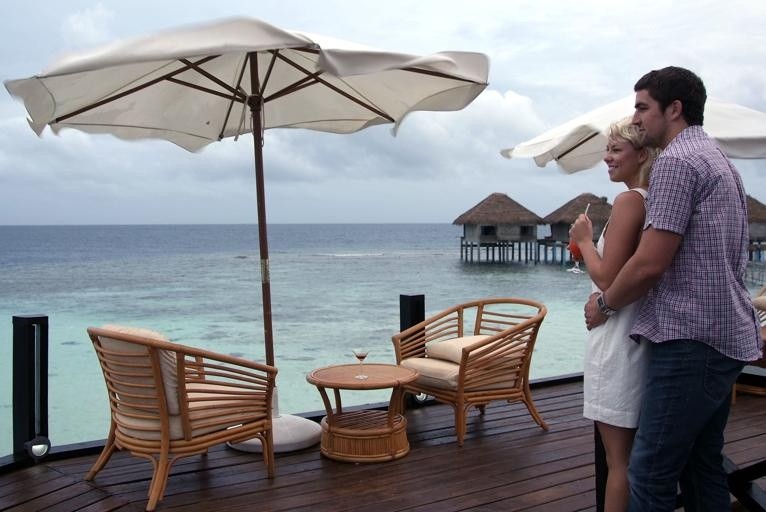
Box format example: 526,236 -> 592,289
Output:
87,326 -> 279,511
392,297 -> 549,448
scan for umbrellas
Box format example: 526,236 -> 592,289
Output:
2,14 -> 488,385
499,91 -> 766,174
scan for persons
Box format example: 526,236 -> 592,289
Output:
571,115 -> 659,510
584,67 -> 763,510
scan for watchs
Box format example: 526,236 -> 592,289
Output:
594,291 -> 622,316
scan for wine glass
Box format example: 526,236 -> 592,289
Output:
566,225 -> 587,276
353,347 -> 370,380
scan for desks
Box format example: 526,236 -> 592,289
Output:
306,363 -> 420,463
